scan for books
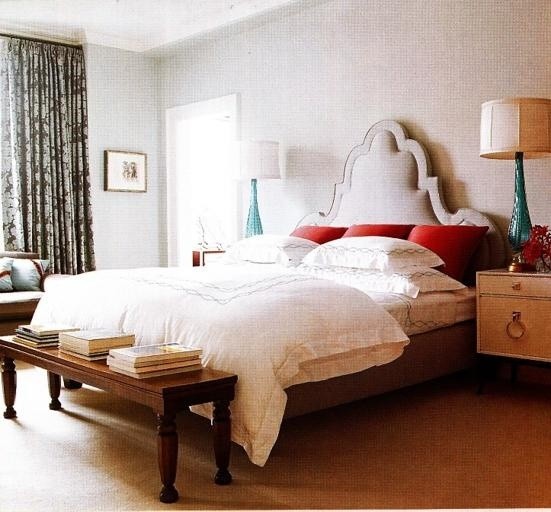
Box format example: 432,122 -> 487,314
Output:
11,323 -> 204,381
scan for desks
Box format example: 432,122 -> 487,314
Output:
192,247 -> 225,268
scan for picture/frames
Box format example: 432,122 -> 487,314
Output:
103,150 -> 149,193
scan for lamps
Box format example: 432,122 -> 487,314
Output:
479,95 -> 551,272
220,136 -> 283,239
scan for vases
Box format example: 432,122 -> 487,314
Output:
535,255 -> 550,275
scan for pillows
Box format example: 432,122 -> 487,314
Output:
300,232 -> 450,274
287,225 -> 348,244
222,233 -> 323,269
291,262 -> 468,299
343,221 -> 416,244
9,258 -> 53,290
408,219 -> 491,285
0,258 -> 15,291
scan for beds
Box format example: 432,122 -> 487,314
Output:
23,117 -> 508,469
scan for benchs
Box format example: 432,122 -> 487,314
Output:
0,333 -> 239,504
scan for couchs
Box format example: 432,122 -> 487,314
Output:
0,249 -> 56,326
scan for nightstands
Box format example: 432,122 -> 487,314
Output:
475,266 -> 551,398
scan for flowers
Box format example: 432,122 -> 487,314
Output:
525,221 -> 550,275
